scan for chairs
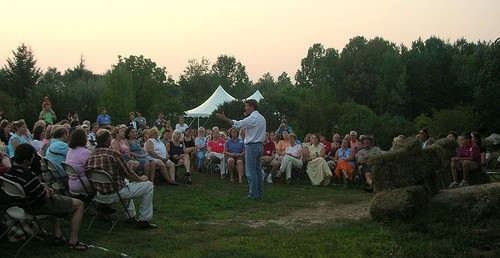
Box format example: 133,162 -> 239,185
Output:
62,161 -> 101,220
44,158 -> 69,195
85,170 -> 133,233
0,176 -> 52,258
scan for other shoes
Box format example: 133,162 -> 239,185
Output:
152,163 -> 474,194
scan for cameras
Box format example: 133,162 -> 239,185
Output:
282,123 -> 285,126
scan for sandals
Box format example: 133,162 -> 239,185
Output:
50,234 -> 89,250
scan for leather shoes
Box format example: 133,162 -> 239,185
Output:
243,193 -> 265,200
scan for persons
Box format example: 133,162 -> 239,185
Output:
66,129 -> 118,215
86,129 -> 158,230
0,94 -> 486,200
0,143 -> 91,251
